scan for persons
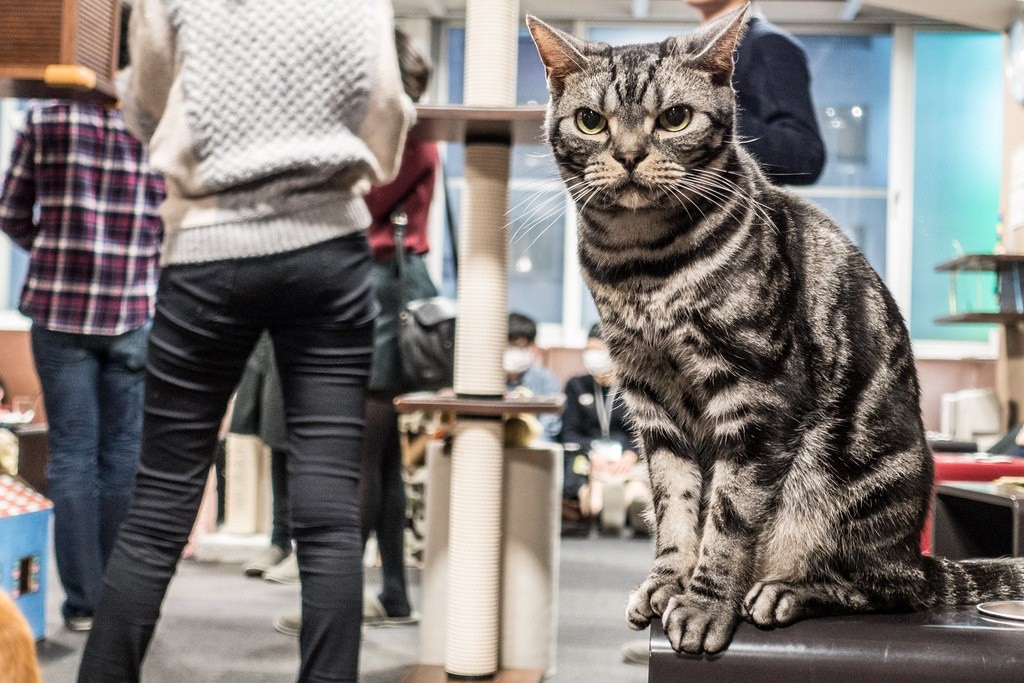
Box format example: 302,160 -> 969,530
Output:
553,323 -> 648,538
617,1 -> 828,662
505,313 -> 564,440
1,102 -> 166,631
275,29 -> 437,637
228,329 -> 302,584
78,0 -> 417,683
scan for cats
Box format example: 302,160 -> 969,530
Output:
526,1 -> 1024,658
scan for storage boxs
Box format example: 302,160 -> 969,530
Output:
1,476 -> 55,643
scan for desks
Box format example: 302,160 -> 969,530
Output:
648,452 -> 1024,683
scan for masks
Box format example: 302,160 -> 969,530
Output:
581,349 -> 618,378
503,344 -> 535,374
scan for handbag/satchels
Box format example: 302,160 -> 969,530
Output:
397,296 -> 457,392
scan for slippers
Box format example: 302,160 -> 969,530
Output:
361,594 -> 420,626
274,610 -> 301,636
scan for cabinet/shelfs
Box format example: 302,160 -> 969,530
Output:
933,255 -> 1024,357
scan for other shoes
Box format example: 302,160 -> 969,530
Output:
245,543 -> 292,577
262,552 -> 300,582
63,614 -> 94,632
